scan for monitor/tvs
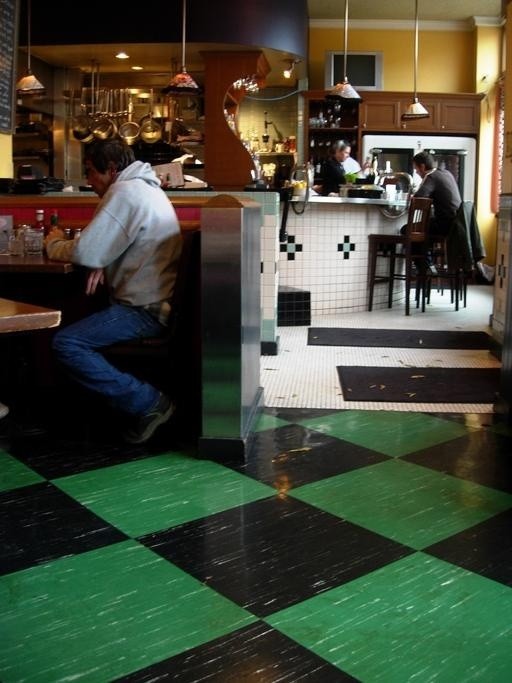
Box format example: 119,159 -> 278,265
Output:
254,152 -> 295,185
325,50 -> 383,92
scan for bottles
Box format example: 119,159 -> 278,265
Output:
73,228 -> 82,239
239,134 -> 296,153
308,99 -> 344,129
64,228 -> 72,240
44,214 -> 64,242
309,134 -> 358,176
33,209 -> 47,251
363,155 -> 378,171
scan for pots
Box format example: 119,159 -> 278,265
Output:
69,87 -> 164,147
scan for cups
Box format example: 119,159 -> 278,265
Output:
0,177 -> 65,195
23,229 -> 44,256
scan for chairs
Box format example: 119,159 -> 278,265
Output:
367,196 -> 476,314
69,226 -> 198,438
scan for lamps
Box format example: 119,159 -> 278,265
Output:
402,0 -> 431,120
15,0 -> 47,91
325,0 -> 363,103
162,0 -> 203,94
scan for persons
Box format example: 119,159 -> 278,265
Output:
399,150 -> 462,275
320,138 -> 375,195
41,133 -> 187,445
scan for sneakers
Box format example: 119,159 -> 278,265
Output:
126,390 -> 178,443
